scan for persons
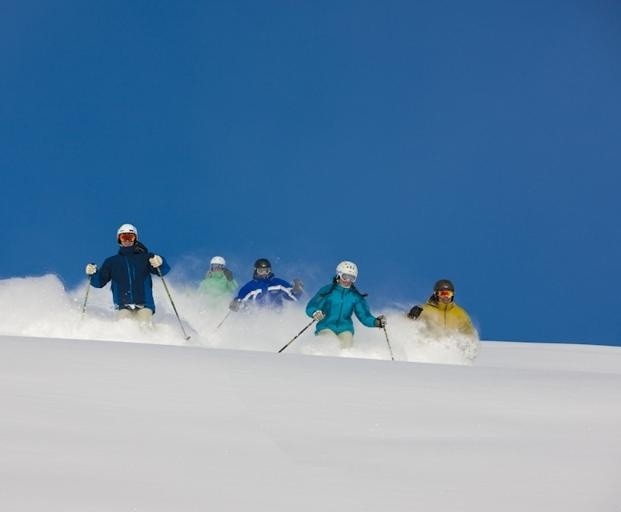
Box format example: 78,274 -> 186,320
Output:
197,256 -> 239,296
408,280 -> 475,336
307,261 -> 387,348
230,258 -> 303,316
86,223 -> 170,323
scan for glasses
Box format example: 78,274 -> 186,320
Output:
340,272 -> 357,283
119,233 -> 136,243
211,264 -> 225,271
437,289 -> 454,299
256,267 -> 271,274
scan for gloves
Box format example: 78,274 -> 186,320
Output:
373,314 -> 388,329
407,304 -> 424,322
312,309 -> 327,322
148,255 -> 164,268
291,278 -> 305,295
228,296 -> 249,313
85,263 -> 98,276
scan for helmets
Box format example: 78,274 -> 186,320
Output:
252,258 -> 271,279
334,259 -> 358,288
115,223 -> 137,247
432,278 -> 456,304
209,255 -> 225,274
